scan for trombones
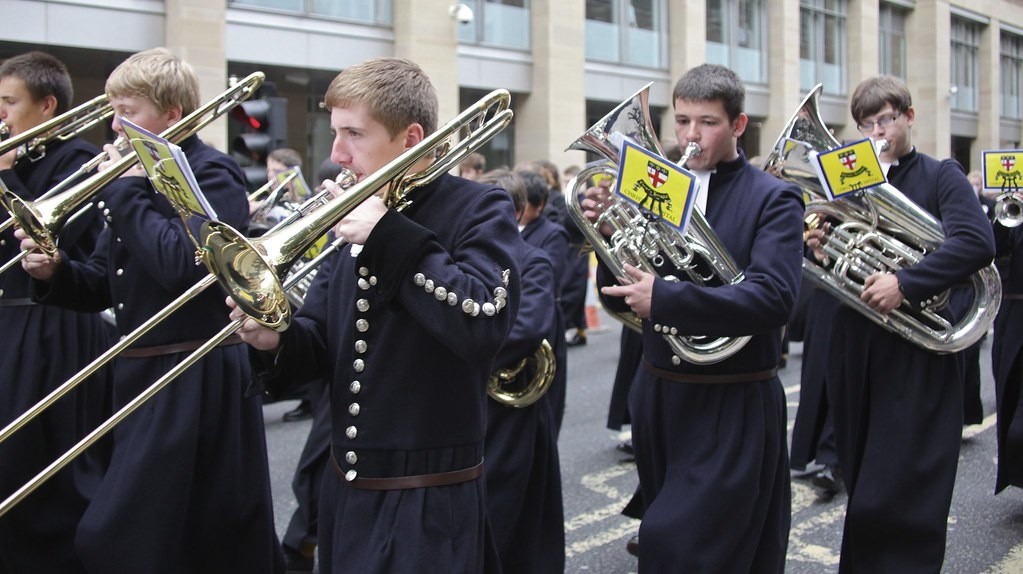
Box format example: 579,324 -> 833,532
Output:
0,93 -> 115,163
0,70 -> 266,275
0,88 -> 514,518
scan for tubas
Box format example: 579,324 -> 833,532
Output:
563,79 -> 755,367
759,82 -> 1004,357
281,258 -> 558,411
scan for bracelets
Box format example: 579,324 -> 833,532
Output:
898,281 -> 905,293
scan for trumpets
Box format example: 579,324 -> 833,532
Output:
992,190 -> 1023,228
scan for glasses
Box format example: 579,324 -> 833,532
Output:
857,110 -> 906,133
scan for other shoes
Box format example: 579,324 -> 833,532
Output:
566,333 -> 585,346
814,472 -> 834,500
626,536 -> 641,556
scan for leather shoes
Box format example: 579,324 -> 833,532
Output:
283,401 -> 313,421
618,441 -> 635,455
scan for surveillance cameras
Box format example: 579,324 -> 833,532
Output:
456,7 -> 474,25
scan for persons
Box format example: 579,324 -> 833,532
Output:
803,75 -> 994,574
581,63 -> 808,574
963,170 -> 1023,496
0,54 -> 116,574
225,57 -> 523,574
462,142 -> 695,556
752,158 -> 843,488
247,149 -> 343,424
14,47 -> 294,574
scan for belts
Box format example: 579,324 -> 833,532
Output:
329,443 -> 484,490
642,357 -> 779,383
119,334 -> 244,358
0,299 -> 38,307
1004,294 -> 1023,300
